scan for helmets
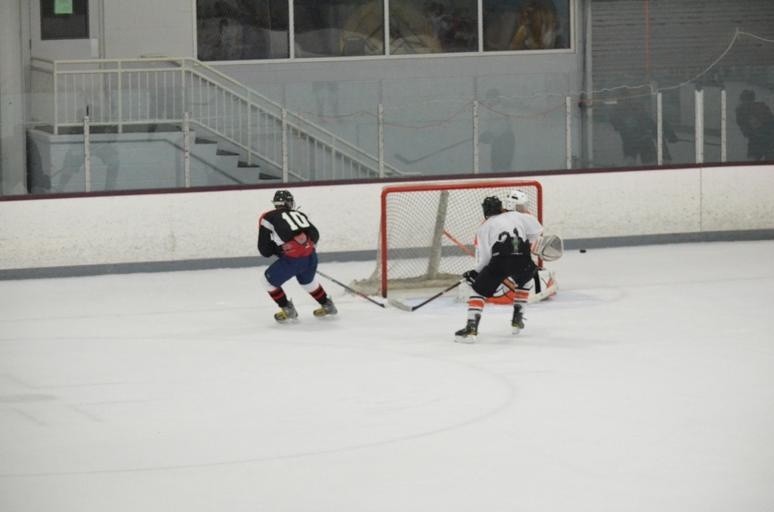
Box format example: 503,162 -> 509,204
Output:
273,191 -> 294,210
481,196 -> 503,220
505,191 -> 527,210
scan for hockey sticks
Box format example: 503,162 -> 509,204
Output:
315,269 -> 391,308
388,278 -> 467,313
442,229 -> 557,306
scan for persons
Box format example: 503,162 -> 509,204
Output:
257,190 -> 337,322
474,188 -> 558,306
451,195 -> 544,338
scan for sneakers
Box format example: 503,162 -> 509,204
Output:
456,314 -> 481,336
512,304 -> 525,328
275,298 -> 297,321
314,297 -> 337,316
